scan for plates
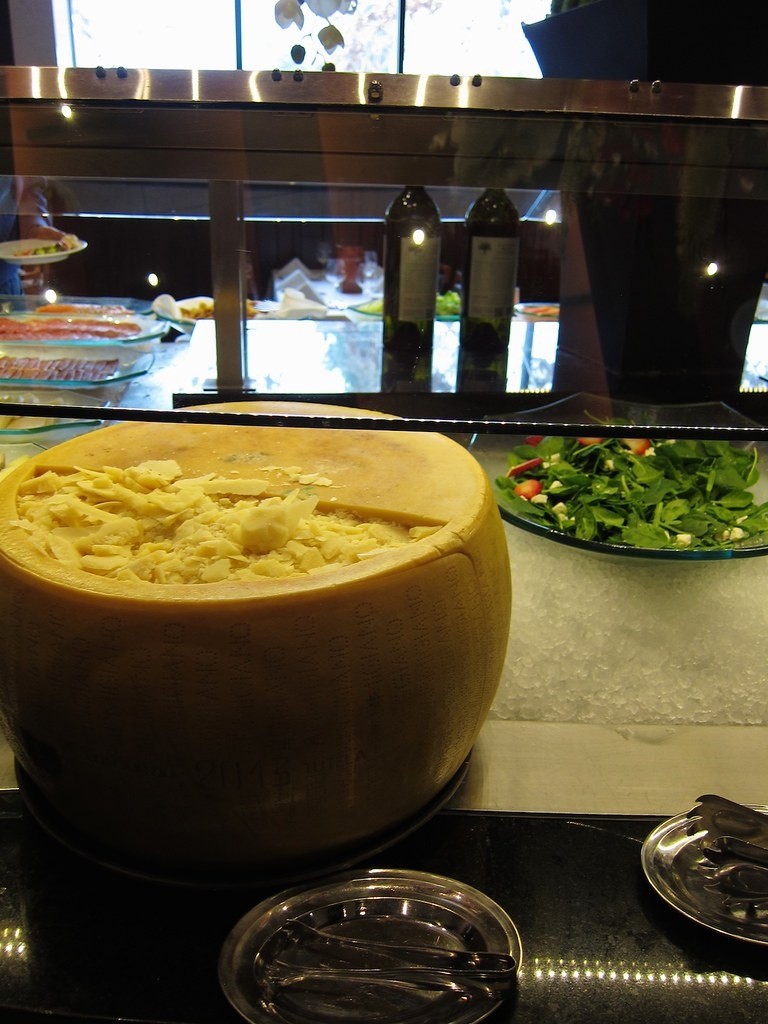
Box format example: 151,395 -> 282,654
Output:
151,295 -> 276,334
219,866 -> 523,1024
0,237 -> 88,264
0,312 -> 171,432
345,296 -> 464,323
512,301 -> 561,323
642,807 -> 768,949
461,390 -> 768,561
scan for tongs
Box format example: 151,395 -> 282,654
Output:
687,793 -> 768,901
262,917 -> 518,992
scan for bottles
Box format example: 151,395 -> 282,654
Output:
458,188 -> 517,352
380,177 -> 438,351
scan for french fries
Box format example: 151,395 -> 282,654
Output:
179,298 -> 259,318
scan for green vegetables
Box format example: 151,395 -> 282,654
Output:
491,410 -> 768,549
436,289 -> 460,316
359,299 -> 384,313
